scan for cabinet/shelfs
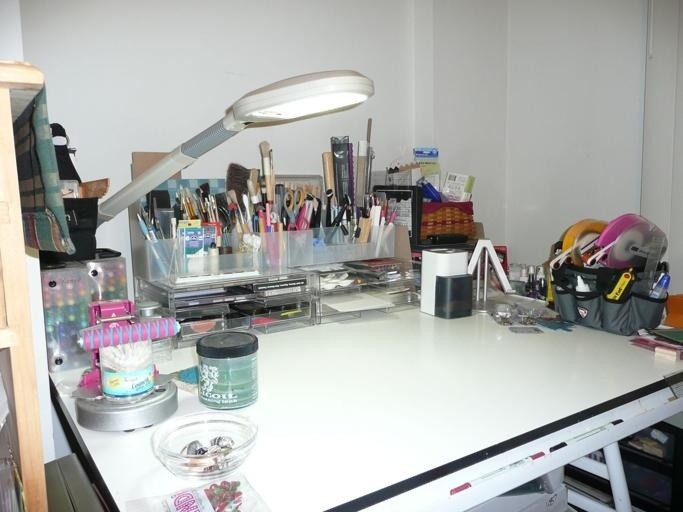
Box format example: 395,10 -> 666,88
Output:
1,61 -> 48,512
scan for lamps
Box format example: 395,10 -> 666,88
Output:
95,67 -> 374,229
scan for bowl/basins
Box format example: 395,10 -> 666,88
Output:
149,413 -> 256,481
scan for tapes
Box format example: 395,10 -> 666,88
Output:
562,214 -> 650,270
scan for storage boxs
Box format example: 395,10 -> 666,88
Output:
39,196 -> 99,268
550,420 -> 682,512
43,255 -> 93,374
82,257 -> 128,302
464,480 -> 568,512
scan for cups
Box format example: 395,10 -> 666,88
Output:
99,336 -> 156,405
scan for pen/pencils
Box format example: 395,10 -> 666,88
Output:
369,192 -> 397,258
175,183 -> 218,223
136,207 -> 174,280
254,203 -> 283,272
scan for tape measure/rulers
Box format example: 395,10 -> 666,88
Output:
330,136 -> 367,208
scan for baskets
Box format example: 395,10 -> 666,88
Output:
420,201 -> 476,242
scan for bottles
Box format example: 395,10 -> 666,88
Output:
196,331 -> 258,410
519,265 -> 547,299
575,275 -> 590,293
647,274 -> 671,299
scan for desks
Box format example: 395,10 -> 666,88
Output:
48,278 -> 682,512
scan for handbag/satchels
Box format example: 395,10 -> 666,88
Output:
550,242 -> 669,336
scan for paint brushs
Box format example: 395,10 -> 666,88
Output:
227,188 -> 242,224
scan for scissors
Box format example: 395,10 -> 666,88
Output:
284,188 -> 310,262
